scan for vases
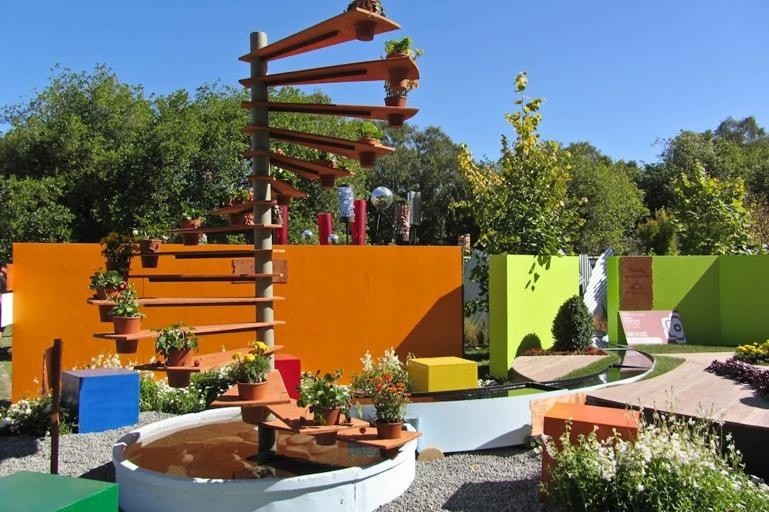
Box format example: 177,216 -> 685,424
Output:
235,379 -> 270,424
315,406 -> 340,446
376,419 -> 402,458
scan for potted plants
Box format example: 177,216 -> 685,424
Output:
357,121 -> 382,168
227,187 -> 249,226
319,150 -> 338,189
383,79 -> 414,128
347,0 -> 384,41
109,283 -> 146,353
272,166 -> 296,206
156,325 -> 196,388
382,37 -> 423,91
176,202 -> 208,245
88,270 -> 126,323
133,216 -> 167,268
100,232 -> 138,284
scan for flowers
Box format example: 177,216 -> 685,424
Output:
296,369 -> 354,424
228,340 -> 272,383
366,372 -> 411,423
